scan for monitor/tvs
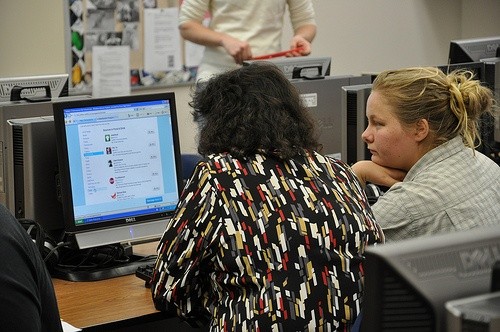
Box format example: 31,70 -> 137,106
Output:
53,92 -> 185,282
243,55 -> 331,81
449,37 -> 500,64
359,223 -> 500,332
372,61 -> 488,156
0,74 -> 71,103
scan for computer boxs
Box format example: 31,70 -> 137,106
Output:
342,83 -> 374,168
4,115 -> 66,244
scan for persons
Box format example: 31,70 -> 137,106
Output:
175,0 -> 318,141
151,60 -> 387,332
0,205 -> 66,332
348,66 -> 500,242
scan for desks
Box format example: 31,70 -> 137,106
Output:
51,241 -> 161,332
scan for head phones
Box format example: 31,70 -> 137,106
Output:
364,184 -> 384,206
19,219 -> 59,266
